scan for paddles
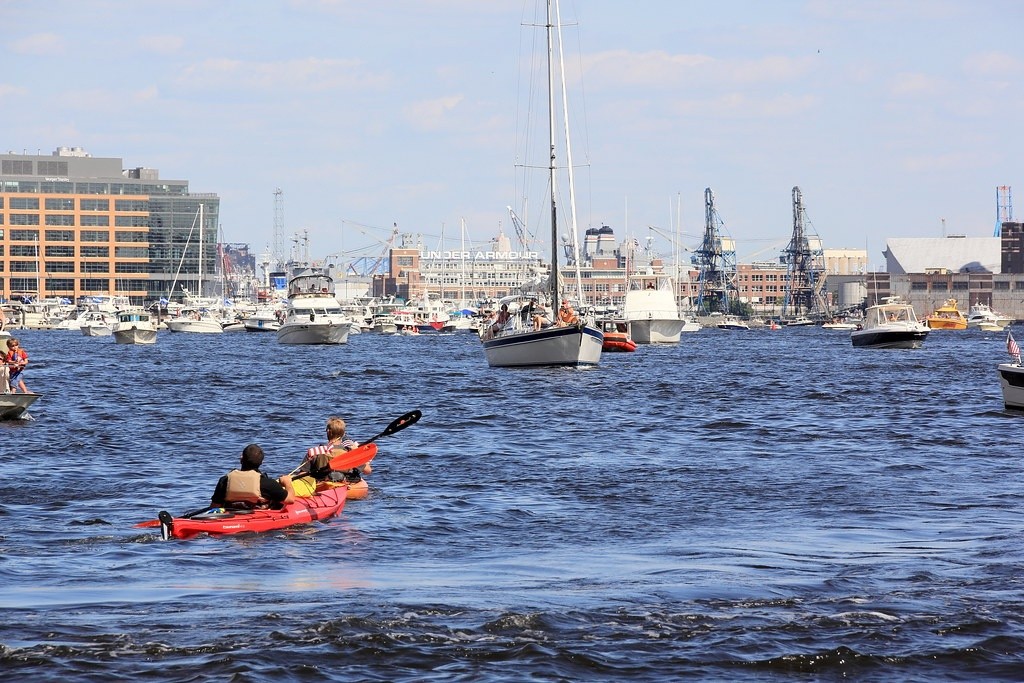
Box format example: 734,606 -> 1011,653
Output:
131,442 -> 379,531
357,409 -> 424,449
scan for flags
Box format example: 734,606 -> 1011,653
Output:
1006,333 -> 1020,360
62,298 -> 71,304
160,297 -> 171,304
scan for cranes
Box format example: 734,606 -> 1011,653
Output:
696,189 -> 742,315
782,186 -> 831,316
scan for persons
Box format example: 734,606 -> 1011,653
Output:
488,302 -> 510,340
274,309 -> 287,323
519,301 -> 537,322
647,282 -> 654,290
301,418 -> 372,480
556,299 -> 580,326
888,313 -> 897,322
309,284 -> 319,292
856,323 -> 863,331
533,302 -> 553,331
209,444 -> 295,508
0,350 -> 6,368
402,324 -> 420,334
6,338 -> 29,393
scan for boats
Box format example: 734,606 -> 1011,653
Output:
851,299 -> 932,349
0,391 -> 43,420
930,300 -> 968,329
786,317 -> 816,327
210,474 -> 368,513
598,316 -> 637,350
767,324 -> 781,330
682,314 -> 702,332
0,200 -> 604,342
822,322 -> 857,331
978,322 -> 1005,332
716,314 -> 750,330
968,303 -> 1017,327
624,280 -> 684,342
159,485 -> 349,539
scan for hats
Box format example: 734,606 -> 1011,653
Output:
562,298 -> 568,304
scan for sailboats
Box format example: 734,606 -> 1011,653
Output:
483,1 -> 603,367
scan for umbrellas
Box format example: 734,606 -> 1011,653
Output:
452,308 -> 477,315
